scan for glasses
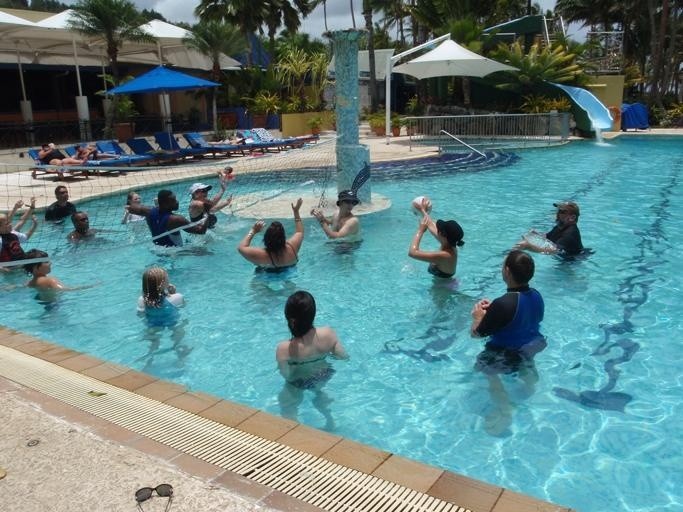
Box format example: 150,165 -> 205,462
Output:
345,200 -> 358,205
135,484 -> 174,512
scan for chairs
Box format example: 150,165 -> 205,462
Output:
27,128 -> 319,181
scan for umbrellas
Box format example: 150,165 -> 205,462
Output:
385,33 -> 520,144
0,8 -> 240,150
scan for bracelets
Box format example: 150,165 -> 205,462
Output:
248,231 -> 254,237
320,218 -> 328,223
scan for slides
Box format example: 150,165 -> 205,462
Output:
543,79 -> 614,132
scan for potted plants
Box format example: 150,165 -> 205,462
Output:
94,74 -> 140,143
366,113 -> 417,137
306,117 -> 322,134
239,89 -> 281,128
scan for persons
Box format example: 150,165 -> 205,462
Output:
313,190 -> 363,255
0,170 -> 232,301
137,268 -> 185,351
470,250 -> 544,372
513,200 -> 582,255
238,198 -> 304,273
275,291 -> 347,431
38,145 -> 87,166
408,199 -> 465,277
74,145 -> 120,159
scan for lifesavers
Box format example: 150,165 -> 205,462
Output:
608,107 -> 621,122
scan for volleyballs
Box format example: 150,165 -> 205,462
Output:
412,196 -> 433,216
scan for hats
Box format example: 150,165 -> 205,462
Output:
553,201 -> 579,214
436,219 -> 463,240
189,183 -> 212,195
336,190 -> 360,206
